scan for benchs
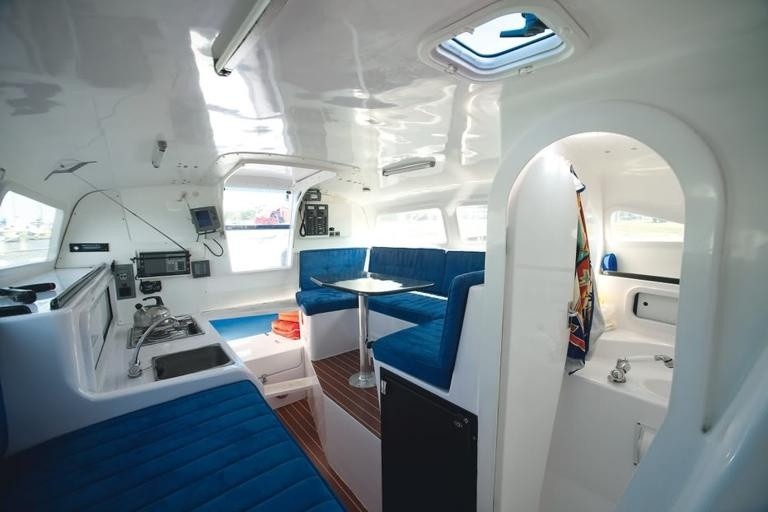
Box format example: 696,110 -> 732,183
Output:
0,376 -> 353,512
292,243 -> 489,413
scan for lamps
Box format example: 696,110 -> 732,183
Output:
209,0 -> 287,80
381,155 -> 437,181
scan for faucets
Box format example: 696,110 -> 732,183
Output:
608,353 -> 673,384
126,316 -> 178,376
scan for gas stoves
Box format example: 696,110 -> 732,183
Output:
125,313 -> 205,349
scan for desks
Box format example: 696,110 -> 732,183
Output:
307,267 -> 436,391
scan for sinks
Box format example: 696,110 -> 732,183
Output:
592,329 -> 673,400
150,343 -> 234,382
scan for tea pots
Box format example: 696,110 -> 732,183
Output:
133,295 -> 169,330
